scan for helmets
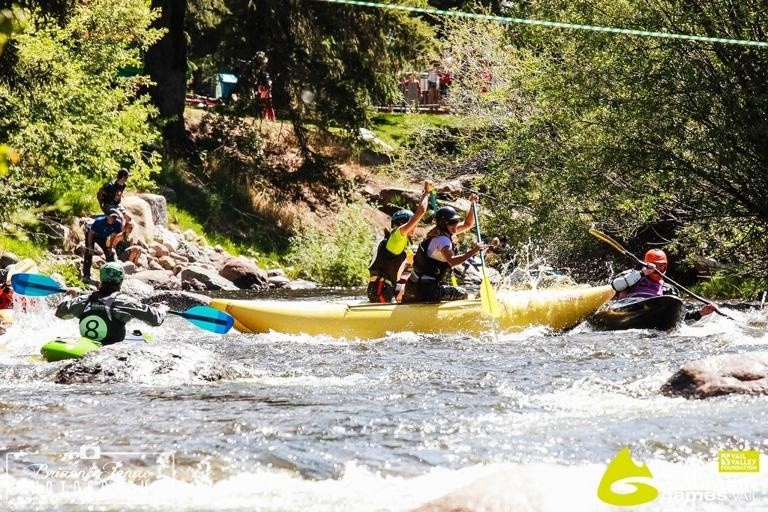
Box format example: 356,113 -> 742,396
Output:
435,206 -> 461,222
391,210 -> 414,228
645,249 -> 667,282
99,262 -> 123,284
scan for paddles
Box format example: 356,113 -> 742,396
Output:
588,229 -> 735,322
11,274 -> 233,334
468,196 -> 499,320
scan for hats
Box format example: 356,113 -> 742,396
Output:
107,210 -> 119,218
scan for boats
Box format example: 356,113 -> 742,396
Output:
207,283 -> 592,335
41,330 -> 149,363
586,294 -> 685,335
225,281 -> 616,345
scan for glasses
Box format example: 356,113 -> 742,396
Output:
446,220 -> 459,226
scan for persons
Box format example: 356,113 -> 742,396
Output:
400,193 -> 485,303
258,71 -> 279,122
611,248 -> 717,327
1,168 -> 170,346
367,178 -> 435,304
394,58 -> 453,117
